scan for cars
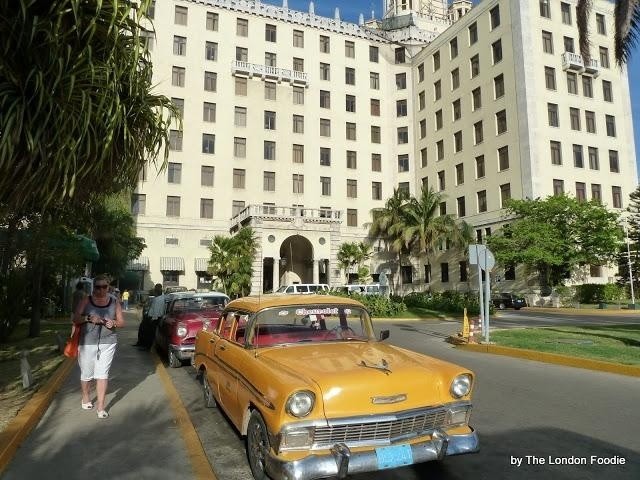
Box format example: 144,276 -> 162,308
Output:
154,283 -> 481,479
492,292 -> 526,310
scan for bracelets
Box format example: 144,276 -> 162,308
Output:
87,315 -> 91,322
112,320 -> 117,328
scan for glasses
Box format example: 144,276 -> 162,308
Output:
94,285 -> 107,290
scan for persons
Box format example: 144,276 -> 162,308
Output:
71,280 -> 85,313
108,287 -> 134,311
134,286 -> 166,352
69,272 -> 123,420
130,283 -> 164,347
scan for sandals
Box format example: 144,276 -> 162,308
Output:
97,409 -> 108,419
81,399 -> 92,410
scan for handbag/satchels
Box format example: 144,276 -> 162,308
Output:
63,324 -> 80,359
